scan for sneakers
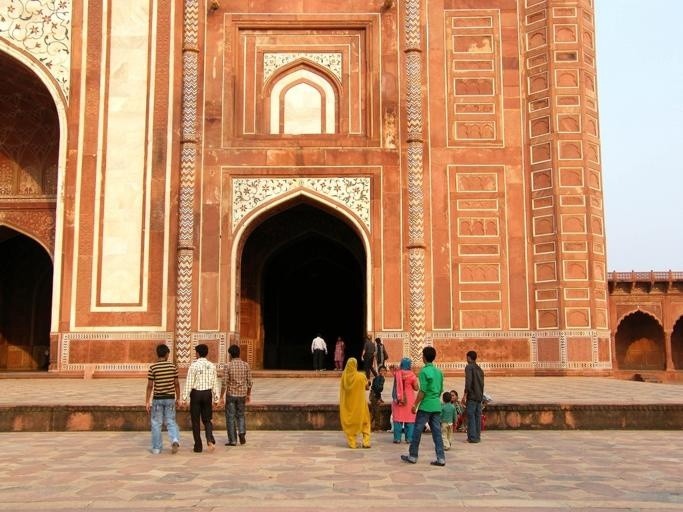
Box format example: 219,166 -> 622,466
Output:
392,440 -> 400,444
400,454 -> 416,464
430,459 -> 446,467
239,433 -> 246,445
225,443 -> 235,446
171,442 -> 178,456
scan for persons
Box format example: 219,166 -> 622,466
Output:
361,335 -> 378,380
369,365 -> 387,430
450,390 -> 464,433
373,337 -> 385,368
439,392 -> 457,451
392,358 -> 419,444
462,351 -> 484,445
217,345 -> 253,446
310,333 -> 328,372
333,337 -> 346,371
181,344 -> 220,453
339,357 -> 371,449
400,347 -> 445,466
145,344 -> 181,455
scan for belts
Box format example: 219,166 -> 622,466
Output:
152,395 -> 175,400
